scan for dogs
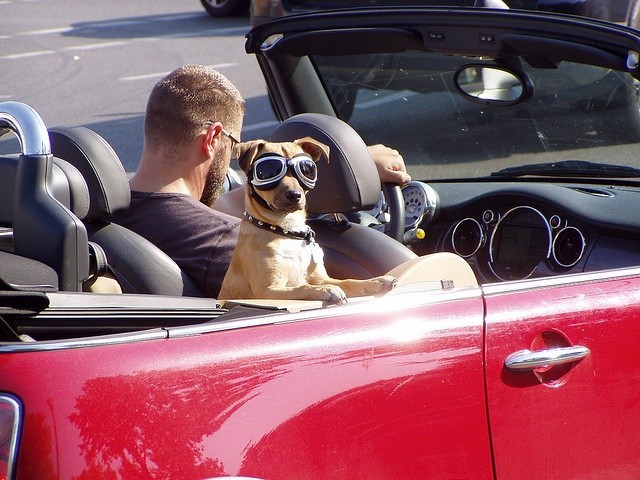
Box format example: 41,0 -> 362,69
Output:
216,135 -> 400,305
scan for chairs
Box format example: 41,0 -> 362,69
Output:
42,122 -> 210,298
262,109 -> 422,281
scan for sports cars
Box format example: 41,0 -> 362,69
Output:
0,7 -> 640,479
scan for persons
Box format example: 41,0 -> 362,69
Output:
110,66 -> 412,298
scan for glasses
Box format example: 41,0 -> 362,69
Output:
204,118 -> 241,148
248,153 -> 318,191
